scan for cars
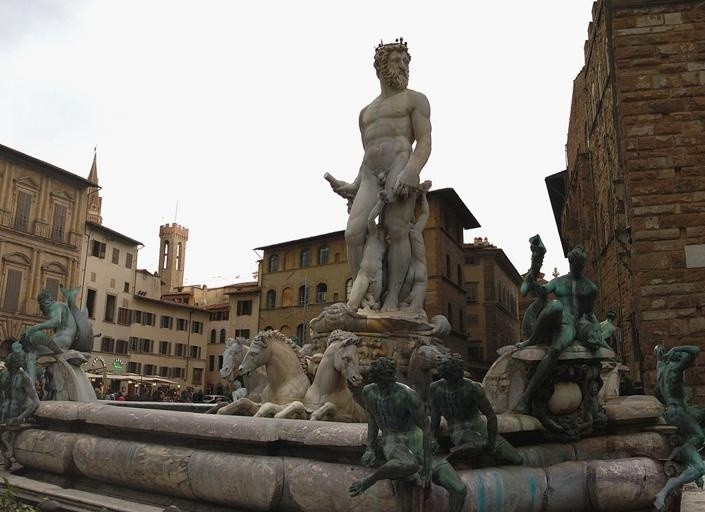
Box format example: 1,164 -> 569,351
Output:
202,394 -> 231,403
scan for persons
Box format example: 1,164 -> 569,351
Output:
230,379 -> 248,402
651,342 -> 705,512
91,381 -> 205,402
1,289 -> 79,470
513,234 -> 600,417
419,354 -> 526,488
346,354 -> 469,510
326,34 -> 433,317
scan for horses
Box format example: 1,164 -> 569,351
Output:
273,329 -> 370,423
204,328 -> 312,417
407,336 -> 451,416
